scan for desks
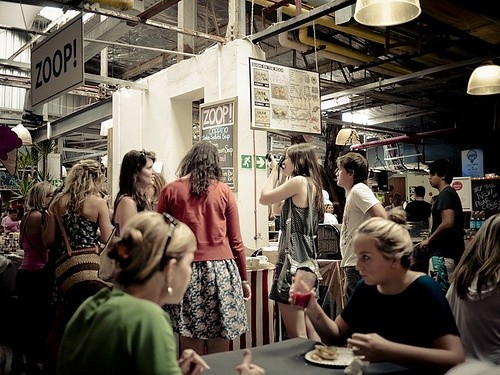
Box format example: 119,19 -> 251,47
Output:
316,258 -> 349,322
198,336 -> 372,375
173,261 -> 276,360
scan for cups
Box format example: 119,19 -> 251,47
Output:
256,255 -> 268,264
246,257 -> 259,267
289,269 -> 317,310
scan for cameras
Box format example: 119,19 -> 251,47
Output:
265,154 -> 285,167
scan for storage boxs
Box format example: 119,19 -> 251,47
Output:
269,220 -> 275,231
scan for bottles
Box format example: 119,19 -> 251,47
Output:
268,221 -> 275,231
275,215 -> 280,231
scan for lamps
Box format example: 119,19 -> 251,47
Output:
353,0 -> 422,28
335,128 -> 362,145
466,60 -> 500,95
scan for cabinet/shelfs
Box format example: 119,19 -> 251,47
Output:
269,230 -> 282,242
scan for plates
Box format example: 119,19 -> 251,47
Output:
305,347 -> 359,365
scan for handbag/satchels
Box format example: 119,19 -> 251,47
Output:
268,251 -> 322,306
55,247 -> 113,301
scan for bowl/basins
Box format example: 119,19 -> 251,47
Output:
3,248 -> 12,253
17,250 -> 24,256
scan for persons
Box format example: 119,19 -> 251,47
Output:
288,208 -> 465,370
111,149 -> 167,238
421,159 -> 465,266
57,212 -> 265,375
0,125 -> 112,375
259,145 -> 325,343
385,185 -> 439,229
336,153 -> 387,301
320,165 -> 338,225
446,214 -> 500,364
156,141 -> 251,355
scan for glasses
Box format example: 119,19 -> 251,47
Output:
140,148 -> 146,164
159,211 -> 180,272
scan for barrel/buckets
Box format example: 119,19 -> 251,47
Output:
261,246 -> 278,264
268,242 -> 278,247
